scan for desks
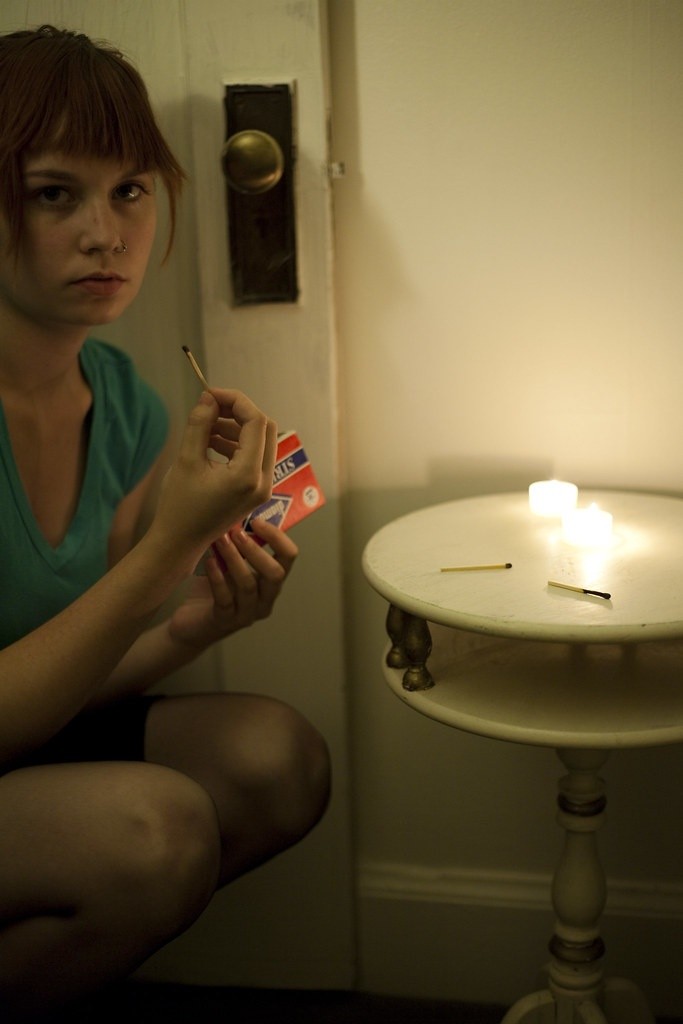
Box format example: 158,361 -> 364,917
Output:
363,487 -> 682,1024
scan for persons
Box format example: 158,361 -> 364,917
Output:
0,28 -> 332,1024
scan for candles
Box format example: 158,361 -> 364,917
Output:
529,479 -> 611,545
